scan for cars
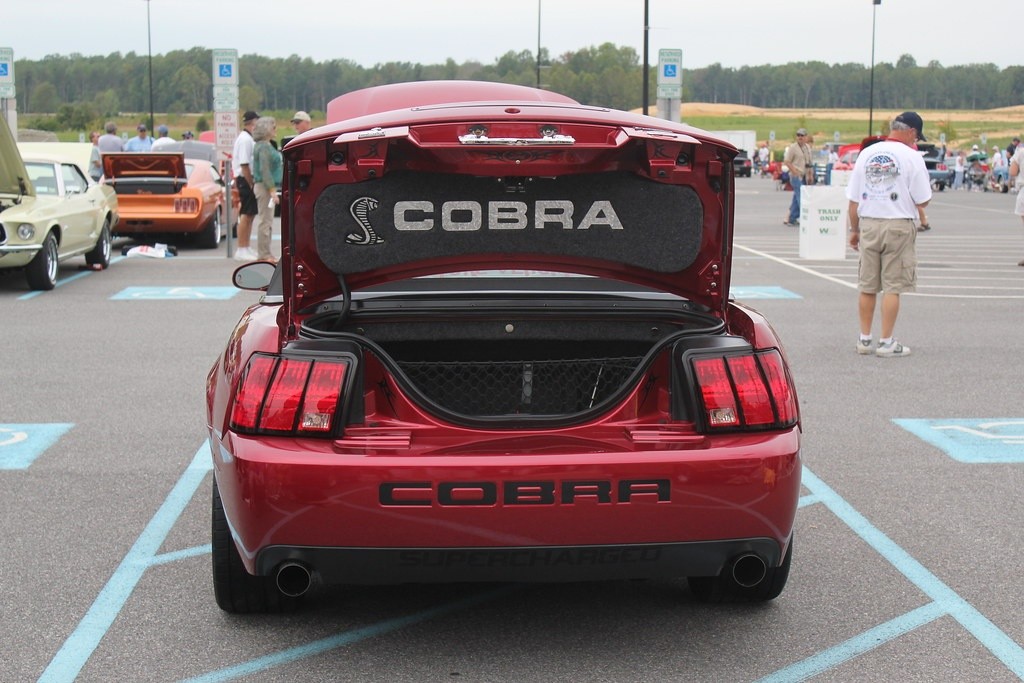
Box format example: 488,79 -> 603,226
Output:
97,130 -> 280,249
733,149 -> 752,177
0,114 -> 121,290
761,134 -> 956,193
205,78 -> 800,619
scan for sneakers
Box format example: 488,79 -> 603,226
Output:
857,335 -> 873,355
877,335 -> 911,358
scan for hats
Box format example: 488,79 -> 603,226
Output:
242,110 -> 260,122
182,131 -> 194,138
158,124 -> 168,133
289,110 -> 311,123
796,128 -> 807,137
894,111 -> 927,142
973,144 -> 979,150
137,124 -> 146,131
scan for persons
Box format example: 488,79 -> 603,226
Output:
123,125 -> 156,152
97,122 -> 125,152
758,140 -> 769,166
947,137 -> 1020,193
290,111 -> 311,134
232,111 -> 260,260
1009,147 -> 1024,267
752,148 -> 760,174
151,125 -> 175,152
783,128 -> 813,226
909,142 -> 931,231
89,131 -> 102,182
252,116 -> 282,263
182,131 -> 194,140
847,111 -> 932,357
820,143 -> 838,185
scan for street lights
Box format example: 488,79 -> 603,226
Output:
867,0 -> 882,136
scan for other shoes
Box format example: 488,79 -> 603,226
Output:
1018,259 -> 1024,267
234,249 -> 258,263
783,219 -> 799,226
257,258 -> 279,265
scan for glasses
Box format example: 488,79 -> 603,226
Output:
796,134 -> 805,137
139,130 -> 146,133
293,119 -> 301,124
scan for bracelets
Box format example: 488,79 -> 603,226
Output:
849,227 -> 859,233
